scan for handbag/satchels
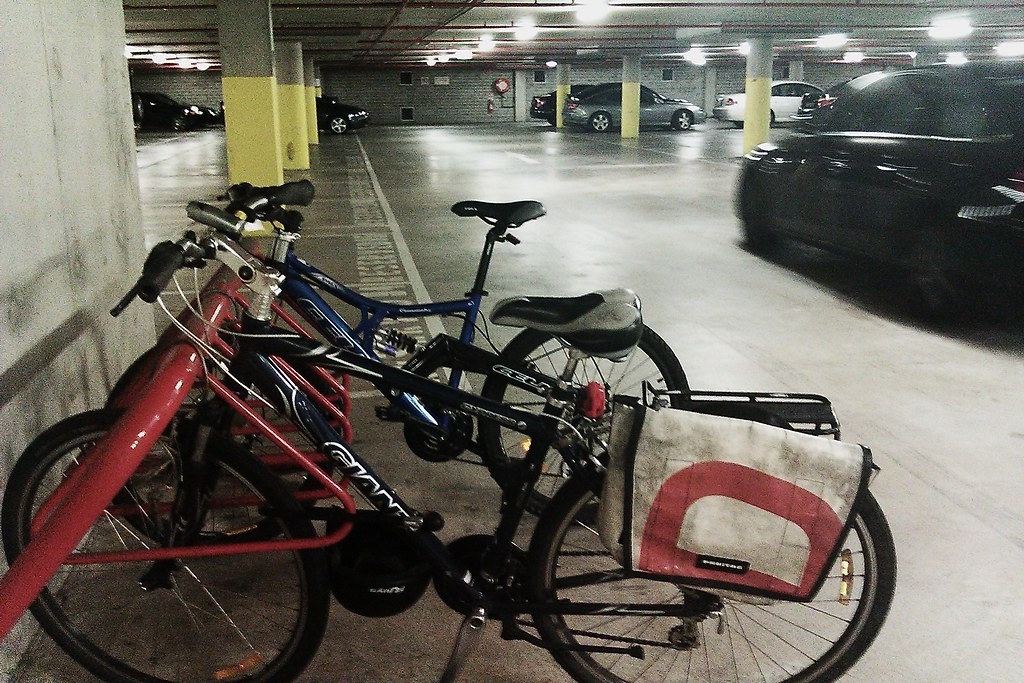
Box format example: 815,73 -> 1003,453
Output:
598,403 -> 873,603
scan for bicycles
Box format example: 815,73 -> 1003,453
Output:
169,179 -> 690,528
0,178 -> 896,682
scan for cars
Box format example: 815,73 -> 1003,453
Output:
732,60 -> 1024,319
529,84 -> 594,125
713,82 -> 822,129
131,92 -> 216,131
561,82 -> 708,134
219,96 -> 369,136
